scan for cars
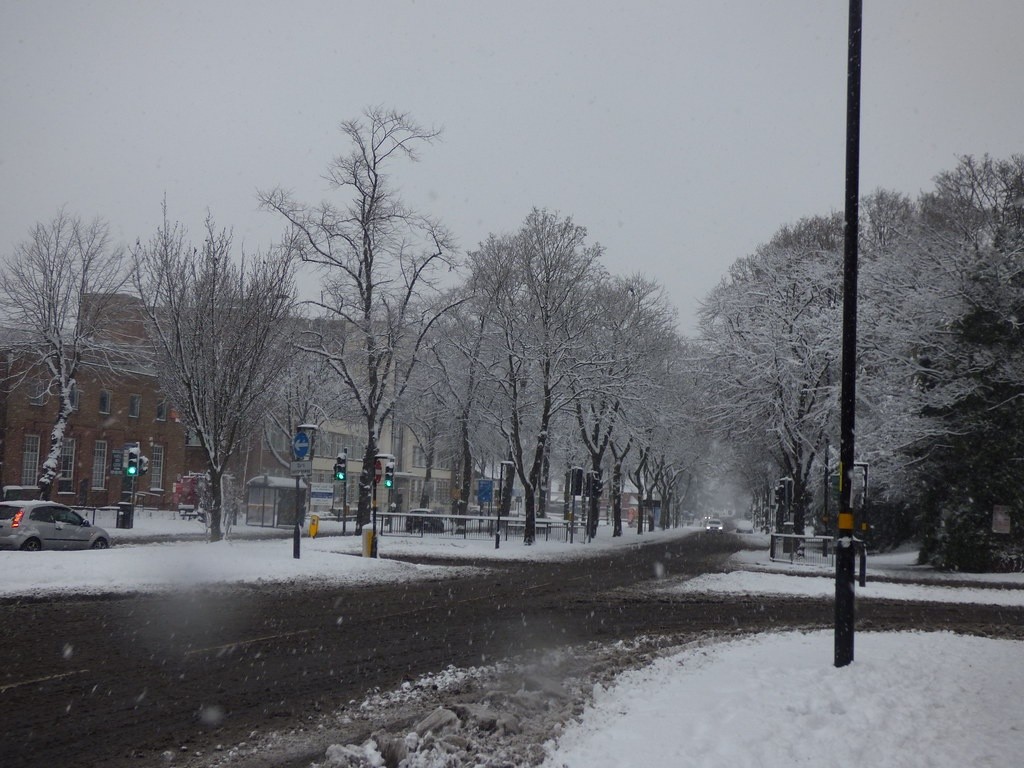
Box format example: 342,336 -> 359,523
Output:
0,500 -> 111,552
405,508 -> 445,533
704,519 -> 724,534
736,523 -> 753,534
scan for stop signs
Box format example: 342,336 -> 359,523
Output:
374,462 -> 382,483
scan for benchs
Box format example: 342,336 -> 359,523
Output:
178,505 -> 199,521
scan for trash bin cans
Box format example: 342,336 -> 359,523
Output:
116,501 -> 133,529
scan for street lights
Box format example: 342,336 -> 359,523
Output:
292,423 -> 318,559
494,459 -> 514,549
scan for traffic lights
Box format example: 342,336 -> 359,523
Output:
384,462 -> 395,488
335,453 -> 347,480
126,447 -> 139,477
